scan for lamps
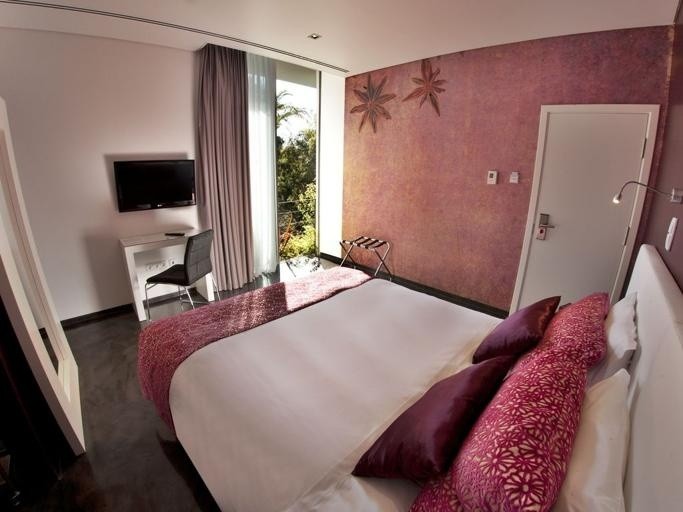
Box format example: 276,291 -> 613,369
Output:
613,178 -> 682,205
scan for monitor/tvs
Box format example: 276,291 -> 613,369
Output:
113,159 -> 197,213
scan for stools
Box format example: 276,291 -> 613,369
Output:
338,236 -> 391,277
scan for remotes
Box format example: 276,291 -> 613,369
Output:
165,232 -> 185,237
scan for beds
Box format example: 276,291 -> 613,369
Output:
137,243 -> 683,512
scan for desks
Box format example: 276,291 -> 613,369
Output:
118,227 -> 214,323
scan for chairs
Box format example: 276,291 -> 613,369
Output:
144,229 -> 220,323
280,228 -> 298,277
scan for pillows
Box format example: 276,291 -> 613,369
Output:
350,356 -> 515,478
551,368 -> 631,512
503,291 -> 610,379
590,292 -> 638,379
409,339 -> 588,512
472,296 -> 561,364
